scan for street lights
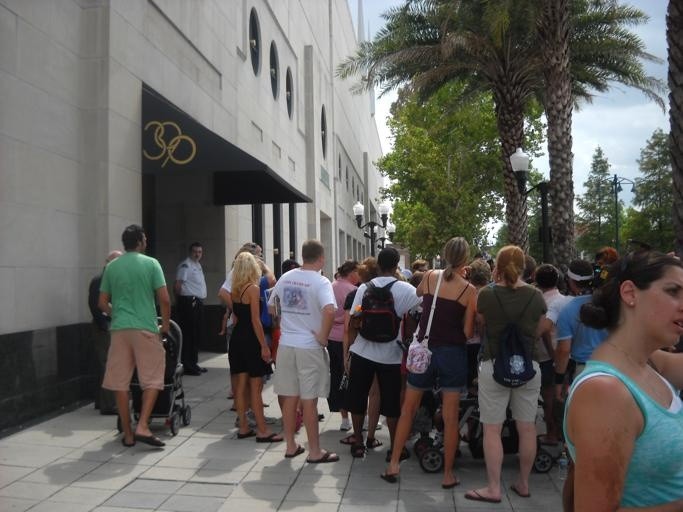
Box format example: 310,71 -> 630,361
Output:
601,175 -> 636,254
510,148 -> 551,265
353,201 -> 390,258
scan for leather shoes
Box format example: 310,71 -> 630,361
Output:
183,366 -> 207,375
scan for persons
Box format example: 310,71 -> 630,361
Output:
172,242 -> 209,376
461,243 -> 549,503
378,236 -> 478,490
96,224 -> 172,447
558,249 -> 683,510
326,235 -> 682,471
87,250 -> 124,415
220,239 -> 341,463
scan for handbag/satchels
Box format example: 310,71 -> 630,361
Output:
406,337 -> 432,373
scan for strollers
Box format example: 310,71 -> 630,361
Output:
114,317 -> 192,435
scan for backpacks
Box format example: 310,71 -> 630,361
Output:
351,280 -> 401,342
488,284 -> 536,387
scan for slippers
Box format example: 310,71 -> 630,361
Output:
122,437 -> 135,447
135,435 -> 165,446
442,476 -> 460,488
511,484 -> 530,497
465,489 -> 501,503
226,397 -> 410,482
